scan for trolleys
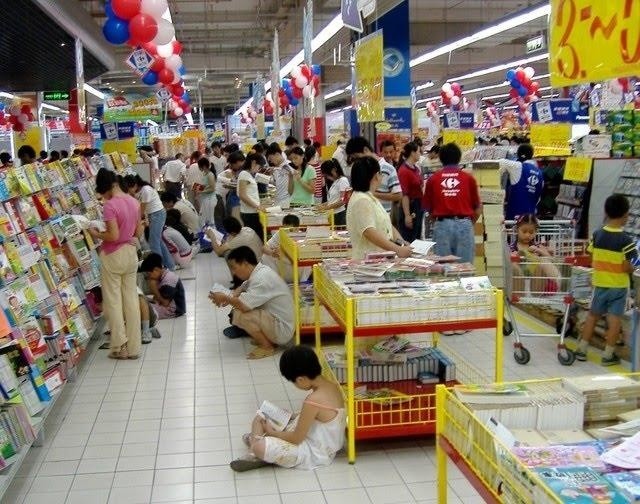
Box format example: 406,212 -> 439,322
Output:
499,218 -> 578,366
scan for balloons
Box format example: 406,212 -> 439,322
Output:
278,61 -> 321,114
238,104 -> 258,125
264,100 -> 274,122
0,100 -> 35,137
505,64 -> 542,128
426,80 -> 468,124
611,77 -> 640,110
481,104 -> 498,125
101,1 -> 190,125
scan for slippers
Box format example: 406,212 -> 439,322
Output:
246,350 -> 275,360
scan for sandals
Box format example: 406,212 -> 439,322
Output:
230,453 -> 259,471
109,352 -> 128,360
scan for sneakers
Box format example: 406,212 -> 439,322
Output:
573,350 -> 589,361
600,354 -> 621,366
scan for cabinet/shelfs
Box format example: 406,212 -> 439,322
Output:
258,204 -> 334,245
312,257 -> 504,465
586,157 -> 640,373
435,371 -> 640,504
278,225 -> 351,347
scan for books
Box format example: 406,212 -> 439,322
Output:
317,239 -> 497,325
0,165 -> 43,204
43,157 -> 71,181
570,265 -> 592,299
615,160 -> 640,253
223,180 -> 239,191
341,188 -> 352,205
288,229 -> 351,259
290,280 -> 313,327
71,155 -> 98,179
257,400 -> 294,432
99,151 -> 132,174
0,181 -> 95,478
323,336 -> 457,385
211,283 -> 232,314
265,207 -> 329,226
203,224 -> 226,248
471,160 -> 506,289
280,163 -> 295,177
190,183 -> 205,192
463,142 -> 518,162
446,372 -> 639,504
254,172 -> 272,185
555,184 -> 583,223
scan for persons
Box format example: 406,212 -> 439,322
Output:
230,345 -> 349,472
574,194 -> 638,368
345,155 -> 413,262
587,128 -> 601,135
160,154 -> 188,202
223,143 -> 232,155
379,138 -> 401,223
214,165 -> 245,229
208,139 -> 228,178
305,146 -> 326,204
138,288 -> 163,345
346,136 -> 403,230
437,136 -> 446,148
164,206 -> 199,248
508,213 -> 554,300
501,144 -> 546,253
139,253 -> 188,319
301,137 -> 312,153
134,145 -> 161,191
125,175 -> 176,271
207,246 -> 296,359
226,149 -> 248,177
312,141 -> 322,153
231,139 -> 241,154
397,142 -> 426,242
0,144 -> 100,167
312,159 -> 351,230
252,138 -> 274,194
425,144 -> 442,172
86,167 -> 144,360
204,214 -> 263,291
283,147 -> 318,210
265,142 -> 296,205
414,137 -> 425,154
244,144 -> 263,169
201,144 -> 216,160
237,152 -> 270,244
421,142 -> 483,336
186,150 -> 204,206
160,191 -> 203,236
286,137 -> 298,156
477,130 -> 531,147
163,225 -> 194,269
195,157 -> 221,226
175,192 -> 199,219
261,214 -> 313,283
